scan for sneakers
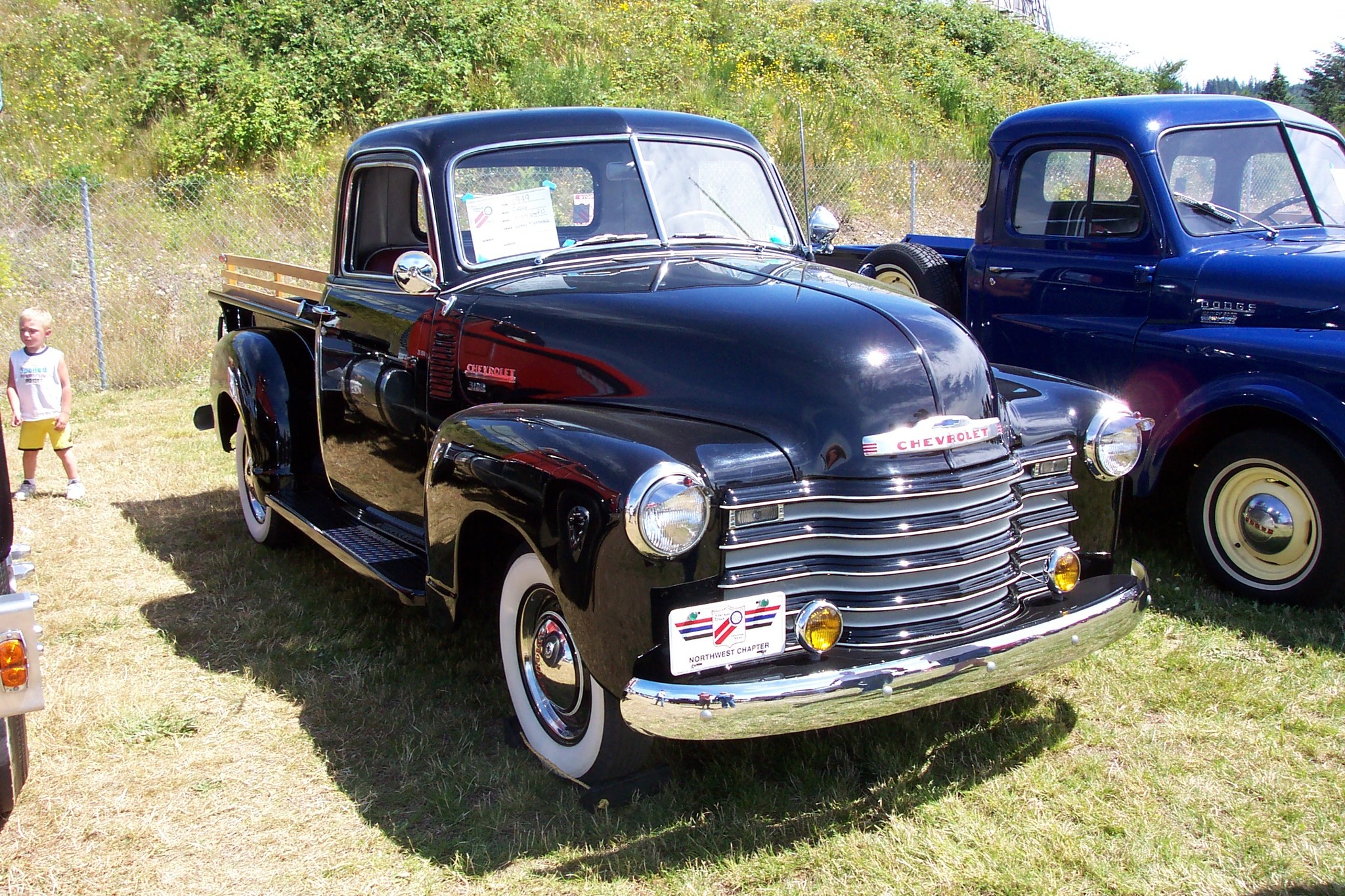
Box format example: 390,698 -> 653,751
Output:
12,480 -> 37,500
65,480 -> 88,500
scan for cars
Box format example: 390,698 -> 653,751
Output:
0,417 -> 47,813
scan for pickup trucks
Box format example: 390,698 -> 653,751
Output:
813,92 -> 1345,606
198,105 -> 1147,787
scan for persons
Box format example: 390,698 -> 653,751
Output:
6,306 -> 86,501
224,356 -> 241,406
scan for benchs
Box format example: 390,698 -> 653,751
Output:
363,245 -> 476,275
